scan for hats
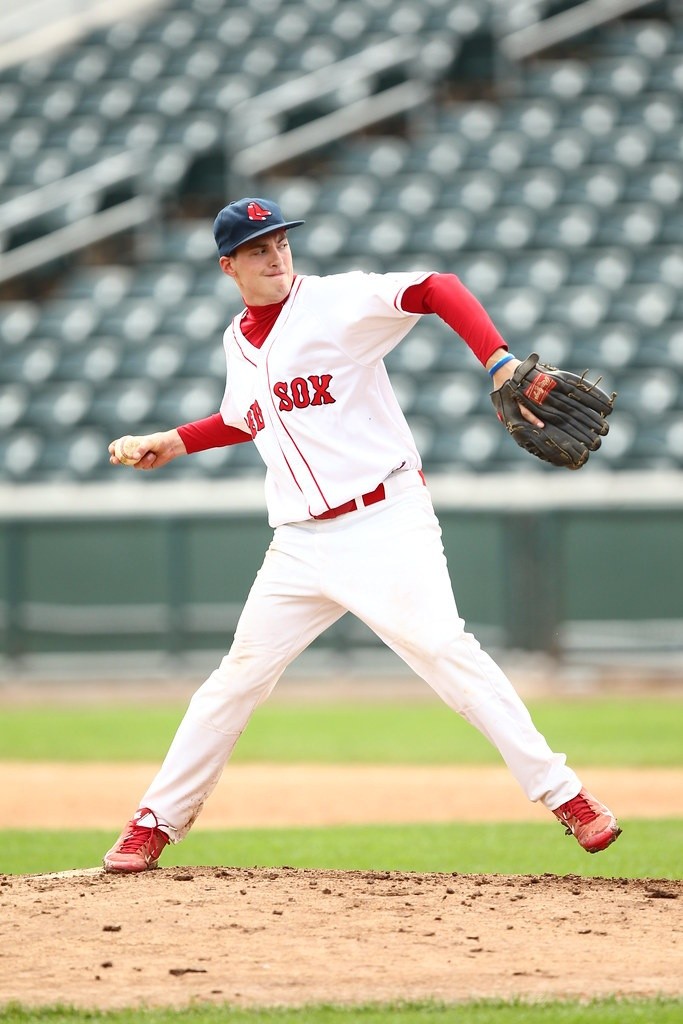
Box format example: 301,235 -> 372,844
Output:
212,197 -> 306,256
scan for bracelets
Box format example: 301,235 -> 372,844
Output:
489,354 -> 516,377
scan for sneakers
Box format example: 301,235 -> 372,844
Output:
103,808 -> 172,875
551,785 -> 621,854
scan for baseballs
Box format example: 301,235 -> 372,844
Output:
115,434 -> 142,465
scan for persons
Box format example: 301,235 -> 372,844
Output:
102,197 -> 623,875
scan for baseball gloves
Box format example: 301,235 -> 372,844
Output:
489,352 -> 618,471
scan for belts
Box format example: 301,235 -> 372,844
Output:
313,470 -> 428,520
